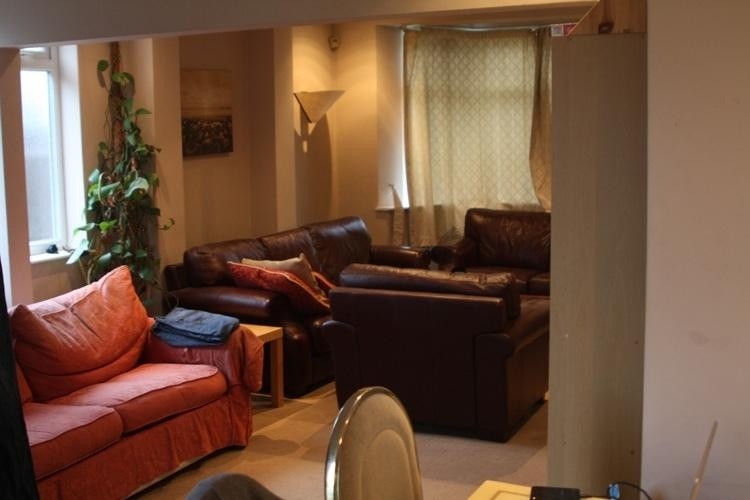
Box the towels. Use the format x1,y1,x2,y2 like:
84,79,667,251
151,307,239,349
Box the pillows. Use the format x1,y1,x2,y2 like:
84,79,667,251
241,251,328,300
225,260,331,315
312,268,337,290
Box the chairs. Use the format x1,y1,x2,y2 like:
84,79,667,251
323,386,424,500
182,471,279,500
313,262,551,444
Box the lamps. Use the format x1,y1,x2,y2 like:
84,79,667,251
294,87,346,122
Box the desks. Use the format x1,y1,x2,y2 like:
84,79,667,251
467,479,532,500
227,322,284,408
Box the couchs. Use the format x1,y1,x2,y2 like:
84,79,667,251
160,214,432,401
434,203,551,295
3,265,265,500
